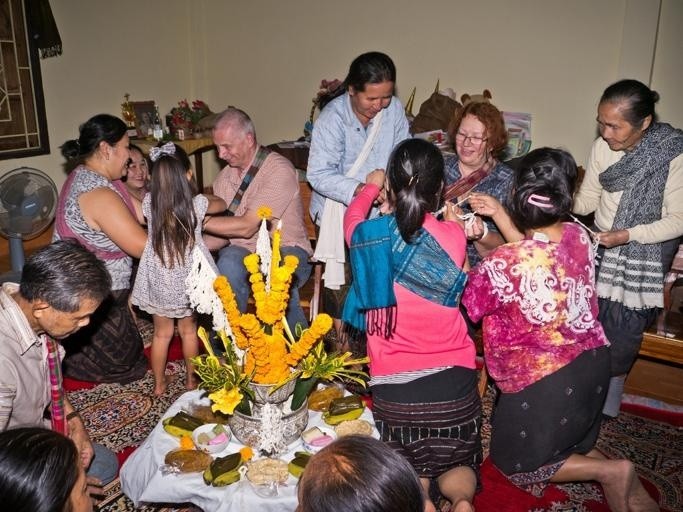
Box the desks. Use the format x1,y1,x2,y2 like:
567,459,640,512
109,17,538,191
130,138,215,194
120,369,382,512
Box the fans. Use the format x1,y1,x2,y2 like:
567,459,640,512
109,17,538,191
0,167,58,286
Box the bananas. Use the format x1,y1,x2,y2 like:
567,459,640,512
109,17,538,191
162,417,208,439
289,451,314,478
203,457,243,486
323,401,366,425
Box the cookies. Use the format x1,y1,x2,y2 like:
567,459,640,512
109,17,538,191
336,420,372,436
245,458,289,485
165,449,214,474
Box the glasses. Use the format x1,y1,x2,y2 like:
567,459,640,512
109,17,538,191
455,132,487,145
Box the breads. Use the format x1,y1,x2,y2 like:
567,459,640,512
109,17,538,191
301,426,323,444
308,386,344,411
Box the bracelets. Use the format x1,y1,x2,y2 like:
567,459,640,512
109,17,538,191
202,214,211,231
66,410,80,421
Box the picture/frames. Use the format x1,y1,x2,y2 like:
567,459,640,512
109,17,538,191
128,100,163,139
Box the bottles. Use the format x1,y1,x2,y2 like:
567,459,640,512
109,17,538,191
151,104,165,142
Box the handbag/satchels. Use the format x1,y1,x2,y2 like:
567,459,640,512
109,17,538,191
410,92,462,136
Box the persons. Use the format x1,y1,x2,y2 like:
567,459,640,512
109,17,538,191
460,148,658,511
200,106,314,369
0,427,93,512
296,434,428,510
344,138,484,511
121,144,179,328
0,237,119,505
52,113,148,385
571,80,682,417
130,141,226,397
306,52,526,393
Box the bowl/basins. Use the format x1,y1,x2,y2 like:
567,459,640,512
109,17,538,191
300,428,338,455
191,422,232,455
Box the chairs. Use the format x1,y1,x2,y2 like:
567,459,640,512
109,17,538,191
468,330,488,398
249,180,323,324
